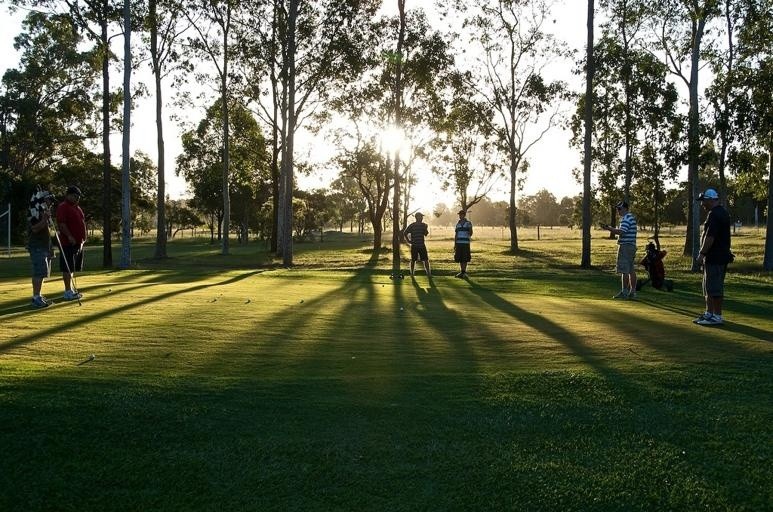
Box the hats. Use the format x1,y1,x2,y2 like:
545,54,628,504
613,201,629,209
415,212,425,217
66,185,85,197
457,210,466,214
695,188,719,201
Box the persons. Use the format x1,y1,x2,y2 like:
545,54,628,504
597,200,640,300
452,210,472,280
21,181,56,308
689,188,737,326
55,186,89,301
403,211,436,277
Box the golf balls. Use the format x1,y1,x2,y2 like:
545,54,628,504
214,298,217,300
109,289,111,291
89,354,94,359
400,307,403,311
301,299,304,302
247,298,251,302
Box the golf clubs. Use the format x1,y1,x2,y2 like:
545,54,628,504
37,183,82,306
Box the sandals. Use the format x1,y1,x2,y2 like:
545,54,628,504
390,273,404,280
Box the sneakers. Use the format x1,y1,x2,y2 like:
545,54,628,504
613,290,635,299
456,272,467,278
692,312,722,326
32,296,49,307
64,290,82,301
